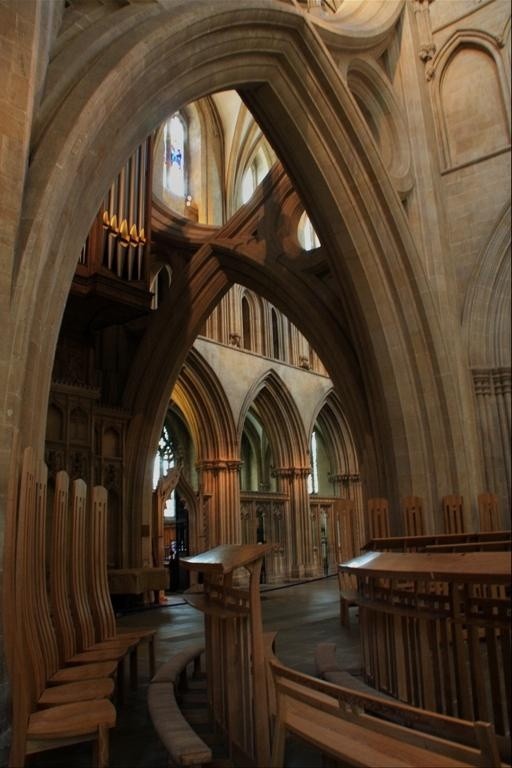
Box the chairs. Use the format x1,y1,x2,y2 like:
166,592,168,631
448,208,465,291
329,500,363,629
15,447,115,700
90,485,157,685
478,493,501,532
55,471,130,694
400,496,426,552
71,478,140,688
35,458,118,686
249,631,501,768
367,497,392,587
443,495,469,534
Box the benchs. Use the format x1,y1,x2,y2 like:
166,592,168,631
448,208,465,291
315,642,339,672
142,644,208,681
147,683,213,767
321,671,403,706
1,441,116,768
